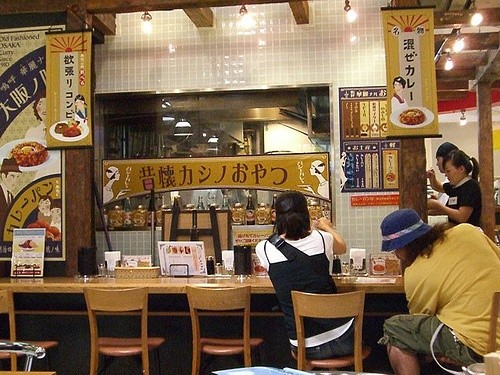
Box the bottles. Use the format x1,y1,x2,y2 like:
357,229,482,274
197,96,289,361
232,203,245,225
342,262,350,275
206,256,214,275
322,204,332,222
255,202,271,225
133,205,146,226
308,202,322,220
196,196,205,210
147,199,157,226
245,194,256,225
220,194,228,210
111,205,124,227
214,263,221,275
332,254,341,274
269,193,277,224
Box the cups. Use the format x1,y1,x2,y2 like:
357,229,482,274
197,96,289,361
78,247,97,277
233,243,252,276
105,251,121,271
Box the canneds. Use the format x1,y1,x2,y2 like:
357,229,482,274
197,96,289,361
307,201,322,227
106,202,274,227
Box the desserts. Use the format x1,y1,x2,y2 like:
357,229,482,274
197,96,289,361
9,141,48,167
399,108,424,125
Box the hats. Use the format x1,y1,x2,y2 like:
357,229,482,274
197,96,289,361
380,208,431,252
436,142,458,157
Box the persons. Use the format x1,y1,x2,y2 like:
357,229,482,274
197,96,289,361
377,208,500,375
255,190,366,361
426,142,483,228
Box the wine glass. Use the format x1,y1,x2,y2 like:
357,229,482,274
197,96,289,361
98,262,106,277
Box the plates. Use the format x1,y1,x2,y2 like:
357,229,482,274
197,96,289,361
390,107,435,128
0,138,59,171
49,119,89,142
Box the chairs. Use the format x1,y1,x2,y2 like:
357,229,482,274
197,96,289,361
427,292,500,375
83,286,166,375
290,290,371,374
0,290,60,372
184,285,264,375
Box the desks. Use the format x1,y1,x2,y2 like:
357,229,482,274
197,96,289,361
0,275,407,317
